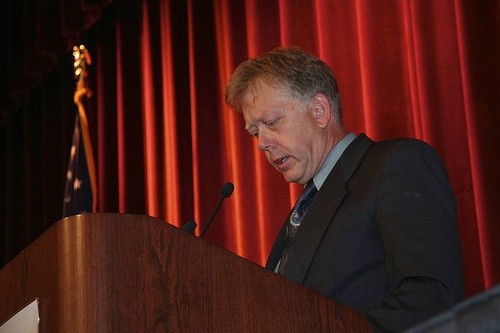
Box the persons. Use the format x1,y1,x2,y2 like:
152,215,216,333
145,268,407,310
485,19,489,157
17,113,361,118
225,46,467,331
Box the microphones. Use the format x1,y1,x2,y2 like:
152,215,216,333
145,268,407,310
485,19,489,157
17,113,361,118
200,181,234,238
180,221,196,233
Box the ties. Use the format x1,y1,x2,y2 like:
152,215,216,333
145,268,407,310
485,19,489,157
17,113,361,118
277,180,318,278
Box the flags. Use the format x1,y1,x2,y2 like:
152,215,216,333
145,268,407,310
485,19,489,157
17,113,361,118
59,109,97,218
